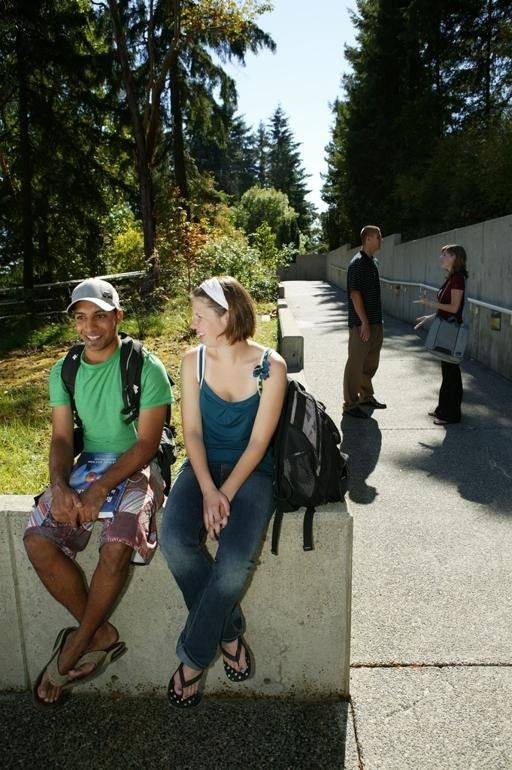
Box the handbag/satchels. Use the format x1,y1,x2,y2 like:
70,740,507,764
425,316,469,368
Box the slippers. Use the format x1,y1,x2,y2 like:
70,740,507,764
64,639,126,692
34,626,80,707
428,410,455,426
166,661,203,709
218,638,253,683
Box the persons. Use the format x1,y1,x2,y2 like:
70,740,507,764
412,245,469,425
23,277,175,708
342,225,386,418
157,274,288,709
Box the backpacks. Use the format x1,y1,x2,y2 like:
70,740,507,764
61,332,179,498
266,376,350,556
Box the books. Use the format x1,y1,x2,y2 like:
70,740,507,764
67,452,130,518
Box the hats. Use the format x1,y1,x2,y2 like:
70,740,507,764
66,278,123,315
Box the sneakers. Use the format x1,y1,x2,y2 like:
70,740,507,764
360,397,387,409
343,401,370,419
